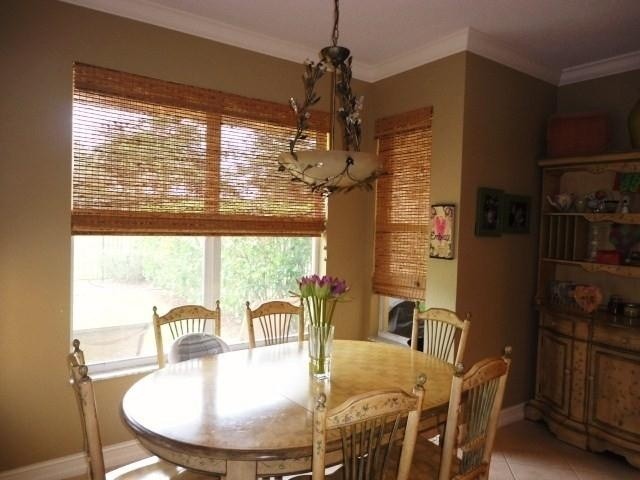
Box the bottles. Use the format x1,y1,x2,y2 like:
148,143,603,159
608,295,639,317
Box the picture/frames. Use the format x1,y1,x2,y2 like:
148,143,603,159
475,187,533,238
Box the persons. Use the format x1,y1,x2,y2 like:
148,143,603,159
511,204,524,232
482,199,497,229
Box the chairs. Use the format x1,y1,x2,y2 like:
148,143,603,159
312,373,427,480
69,338,218,480
410,302,472,368
246,298,304,347
368,345,512,480
151,300,221,369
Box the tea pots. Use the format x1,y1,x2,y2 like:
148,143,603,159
603,199,620,213
546,191,579,213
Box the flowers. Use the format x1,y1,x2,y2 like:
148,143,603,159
290,274,353,375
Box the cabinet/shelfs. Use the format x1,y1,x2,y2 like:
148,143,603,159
524,150,639,469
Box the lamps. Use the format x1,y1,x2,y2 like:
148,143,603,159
273,2,386,199
